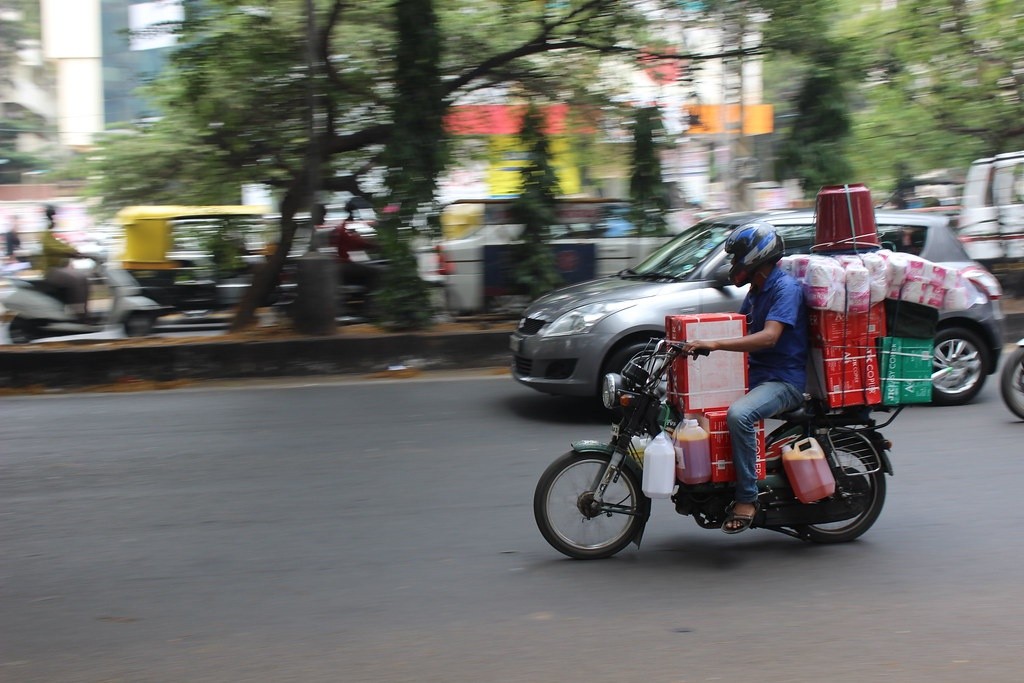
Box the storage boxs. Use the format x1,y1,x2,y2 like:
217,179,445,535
809,300,939,408
666,312,766,482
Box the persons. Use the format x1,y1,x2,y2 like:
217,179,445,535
5,214,21,255
41,204,89,320
302,202,378,264
681,221,810,534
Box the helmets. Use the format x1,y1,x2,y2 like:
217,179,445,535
725,221,785,288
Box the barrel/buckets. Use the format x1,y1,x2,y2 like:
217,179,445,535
672,418,713,481
781,437,835,503
812,182,880,253
641,431,676,499
627,436,652,469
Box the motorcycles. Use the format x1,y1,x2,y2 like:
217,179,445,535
533,335,910,561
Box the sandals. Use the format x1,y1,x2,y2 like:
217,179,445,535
721,498,762,533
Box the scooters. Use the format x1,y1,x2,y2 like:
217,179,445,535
0,238,178,344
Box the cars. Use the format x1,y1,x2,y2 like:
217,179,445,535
508,203,1010,425
